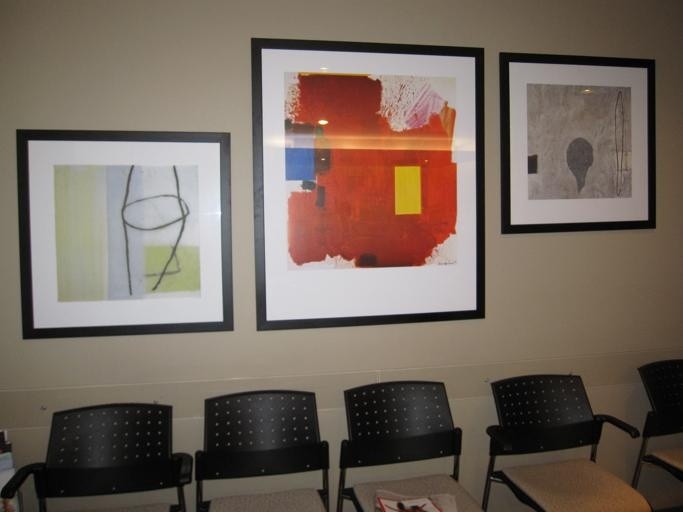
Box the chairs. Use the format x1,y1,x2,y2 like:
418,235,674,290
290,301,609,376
633,359,682,494
2,401,192,512
479,373,655,510
336,380,488,512
194,390,333,510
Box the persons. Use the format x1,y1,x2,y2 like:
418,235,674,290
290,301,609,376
410,503,428,512
385,501,428,512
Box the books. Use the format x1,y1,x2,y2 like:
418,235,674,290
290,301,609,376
375,495,444,512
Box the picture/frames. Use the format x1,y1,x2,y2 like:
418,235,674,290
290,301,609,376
496,48,659,237
13,128,235,339
249,36,488,333
